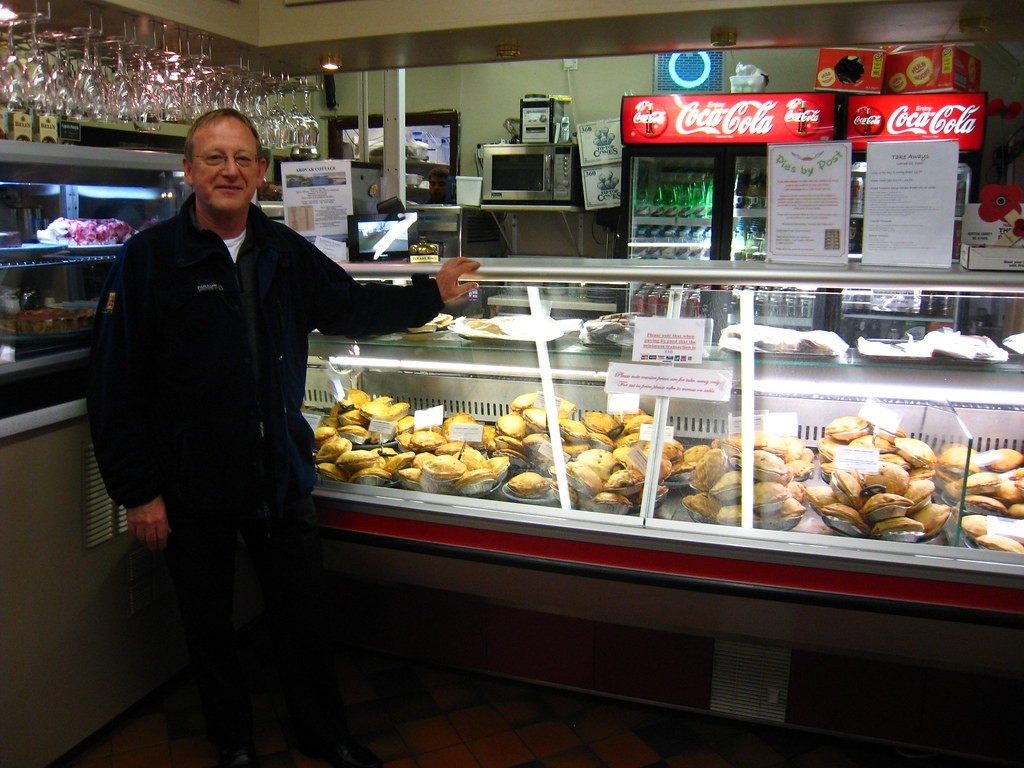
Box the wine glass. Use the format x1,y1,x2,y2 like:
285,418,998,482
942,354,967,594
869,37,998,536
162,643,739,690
0,13,321,150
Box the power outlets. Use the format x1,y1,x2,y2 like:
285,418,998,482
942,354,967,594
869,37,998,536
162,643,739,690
561,58,579,71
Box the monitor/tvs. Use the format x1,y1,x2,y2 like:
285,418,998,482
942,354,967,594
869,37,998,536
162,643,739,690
347,211,418,262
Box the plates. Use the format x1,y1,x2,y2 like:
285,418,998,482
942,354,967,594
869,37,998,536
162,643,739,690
39,240,124,253
0,243,66,261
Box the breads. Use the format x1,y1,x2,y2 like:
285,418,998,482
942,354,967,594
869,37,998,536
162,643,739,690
313,388,1024,554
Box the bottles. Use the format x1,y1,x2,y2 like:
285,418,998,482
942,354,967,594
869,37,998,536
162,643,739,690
634,172,713,218
634,224,712,259
852,294,899,349
732,217,761,261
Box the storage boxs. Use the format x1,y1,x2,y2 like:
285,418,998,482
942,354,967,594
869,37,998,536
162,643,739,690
814,47,888,94
729,75,766,94
887,46,982,96
960,243,1024,271
455,175,483,207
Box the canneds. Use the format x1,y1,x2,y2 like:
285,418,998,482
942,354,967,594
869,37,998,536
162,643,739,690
955,181,966,217
731,286,811,319
851,177,864,214
633,286,699,318
849,220,858,253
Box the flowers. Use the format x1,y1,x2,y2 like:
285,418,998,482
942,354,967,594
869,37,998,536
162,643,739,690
986,99,1022,183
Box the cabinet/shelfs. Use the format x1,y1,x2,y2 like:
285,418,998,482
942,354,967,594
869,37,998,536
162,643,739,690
303,261,1024,768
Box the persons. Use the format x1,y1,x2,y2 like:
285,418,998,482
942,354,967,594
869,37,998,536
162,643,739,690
421,167,447,205
84,109,478,768
899,301,953,341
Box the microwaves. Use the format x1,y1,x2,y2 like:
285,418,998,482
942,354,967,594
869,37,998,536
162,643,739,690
481,140,575,202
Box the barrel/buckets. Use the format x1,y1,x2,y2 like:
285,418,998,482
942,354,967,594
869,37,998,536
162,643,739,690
455,175,483,207
